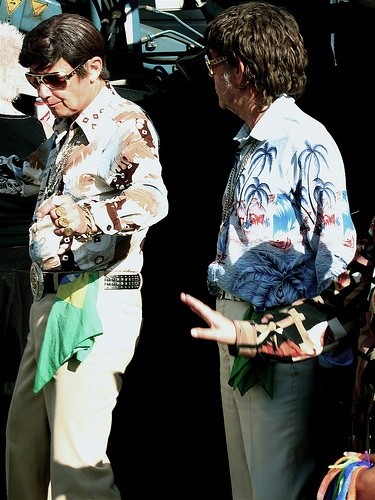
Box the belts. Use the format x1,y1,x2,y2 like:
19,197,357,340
30,262,140,301
218,290,243,301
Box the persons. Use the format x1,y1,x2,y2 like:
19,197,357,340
180,217,375,460
356,454,375,500
204,1,357,500
5,13,170,500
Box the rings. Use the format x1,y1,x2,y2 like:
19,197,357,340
51,195,74,243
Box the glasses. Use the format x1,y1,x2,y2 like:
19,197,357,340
205,54,229,75
25,56,92,91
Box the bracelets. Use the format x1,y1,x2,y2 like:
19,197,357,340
74,204,93,243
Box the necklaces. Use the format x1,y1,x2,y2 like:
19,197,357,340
222,139,260,227
45,126,85,199
